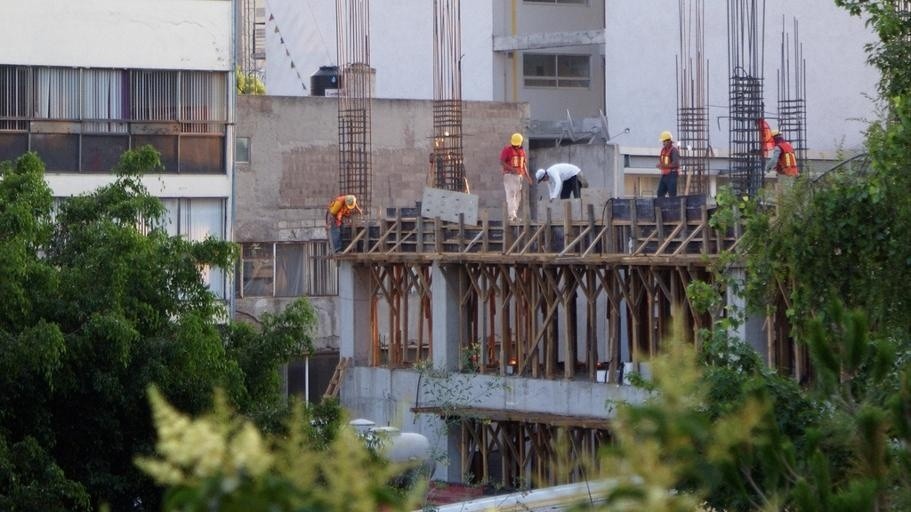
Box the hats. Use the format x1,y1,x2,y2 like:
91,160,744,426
345,193,357,211
771,129,782,136
536,168,546,184
511,133,525,148
659,131,672,141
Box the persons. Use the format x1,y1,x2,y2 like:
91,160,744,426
765,130,797,192
535,163,589,203
501,133,533,224
757,118,775,157
656,132,680,198
325,194,362,255
